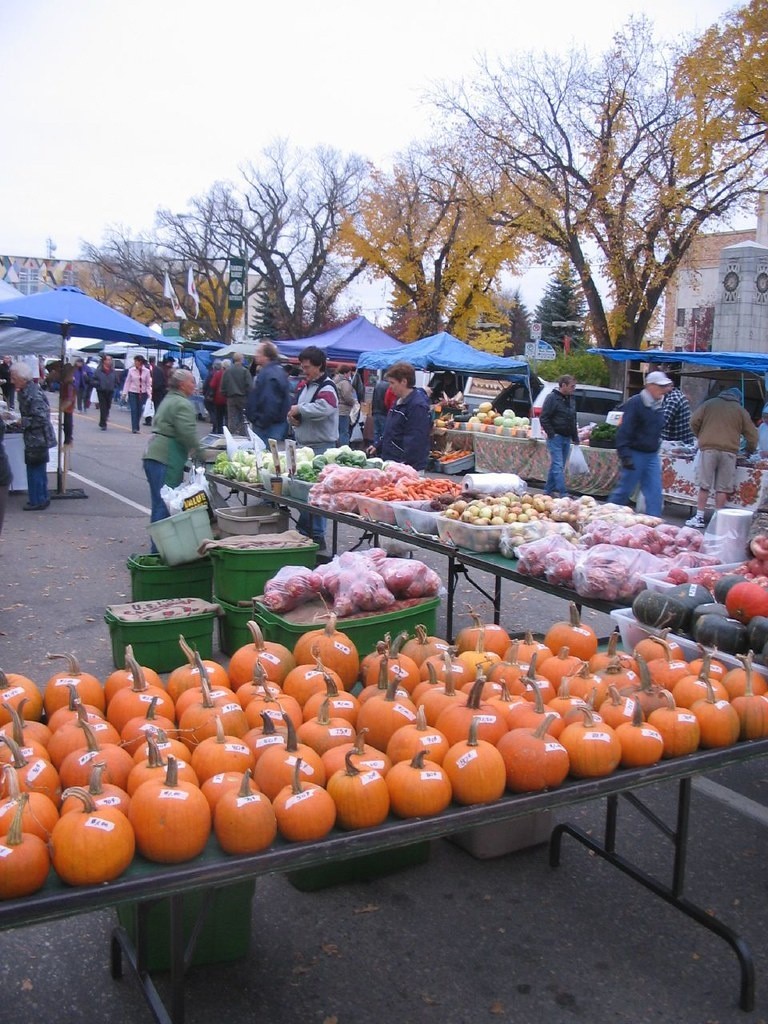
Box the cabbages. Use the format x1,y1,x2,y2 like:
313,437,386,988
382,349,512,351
493,409,529,428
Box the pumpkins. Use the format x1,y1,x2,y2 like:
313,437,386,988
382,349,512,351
0,600,768,901
632,574,768,668
440,450,470,464
454,401,495,425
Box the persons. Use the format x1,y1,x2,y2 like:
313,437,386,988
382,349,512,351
287,346,339,550
756,403,768,458
72,358,91,413
685,388,760,527
6,362,56,510
541,376,579,498
143,357,175,426
203,352,253,434
662,383,694,447
122,355,153,434
60,363,75,450
247,342,291,510
0,356,14,410
333,365,363,450
606,371,672,519
90,355,120,430
365,362,462,474
0,419,13,533
143,369,205,554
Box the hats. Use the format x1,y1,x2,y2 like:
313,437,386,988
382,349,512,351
646,371,672,386
763,406,768,414
730,387,742,401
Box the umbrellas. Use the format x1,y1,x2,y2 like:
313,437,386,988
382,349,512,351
77,323,256,365
588,349,768,397
0,285,182,501
349,332,534,416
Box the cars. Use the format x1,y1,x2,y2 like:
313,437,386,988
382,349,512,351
111,357,125,371
41,357,61,373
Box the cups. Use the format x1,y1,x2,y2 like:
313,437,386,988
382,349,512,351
270,477,283,496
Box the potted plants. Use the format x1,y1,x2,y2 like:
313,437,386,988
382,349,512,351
588,422,619,449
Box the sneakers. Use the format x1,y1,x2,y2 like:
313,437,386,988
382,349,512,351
685,516,706,527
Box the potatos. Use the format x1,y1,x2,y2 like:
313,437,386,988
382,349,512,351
516,520,721,601
663,535,768,595
261,548,442,616
419,491,491,512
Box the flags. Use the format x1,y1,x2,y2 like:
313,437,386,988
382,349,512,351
164,275,188,320
188,269,200,318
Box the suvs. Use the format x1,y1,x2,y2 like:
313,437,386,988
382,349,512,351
489,355,625,431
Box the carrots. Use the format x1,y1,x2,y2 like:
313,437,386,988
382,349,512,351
309,464,463,523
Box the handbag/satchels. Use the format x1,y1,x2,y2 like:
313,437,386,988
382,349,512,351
223,426,266,462
25,415,50,465
370,533,419,553
143,398,155,418
350,422,364,442
636,489,665,514
89,387,99,403
161,466,219,526
569,444,588,476
349,402,360,425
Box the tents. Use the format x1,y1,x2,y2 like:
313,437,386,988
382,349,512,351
276,318,403,362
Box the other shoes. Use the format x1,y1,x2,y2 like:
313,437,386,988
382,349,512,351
558,494,577,500
543,492,559,498
23,500,50,510
388,551,411,559
102,426,106,431
311,536,327,551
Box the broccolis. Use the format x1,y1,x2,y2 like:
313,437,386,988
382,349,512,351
367,457,383,463
222,444,366,482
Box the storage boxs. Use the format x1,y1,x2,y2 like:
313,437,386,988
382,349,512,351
260,469,289,495
434,459,443,472
287,478,317,502
389,503,439,535
351,492,395,525
440,452,475,474
445,811,552,860
103,507,440,663
452,421,525,436
609,608,768,679
640,562,742,594
432,512,502,554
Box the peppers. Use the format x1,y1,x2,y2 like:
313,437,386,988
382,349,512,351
296,452,383,482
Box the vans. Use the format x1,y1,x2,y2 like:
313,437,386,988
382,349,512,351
678,366,768,429
286,355,512,415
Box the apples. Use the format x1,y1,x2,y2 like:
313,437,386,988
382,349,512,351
445,491,663,559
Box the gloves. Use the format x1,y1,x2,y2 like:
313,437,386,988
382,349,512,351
622,457,636,470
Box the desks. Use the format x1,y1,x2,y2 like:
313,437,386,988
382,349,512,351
431,428,768,520
1,412,29,492
45,412,65,473
184,459,640,643
0,736,768,1024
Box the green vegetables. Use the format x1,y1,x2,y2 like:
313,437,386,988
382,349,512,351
590,421,619,440
214,450,228,475
